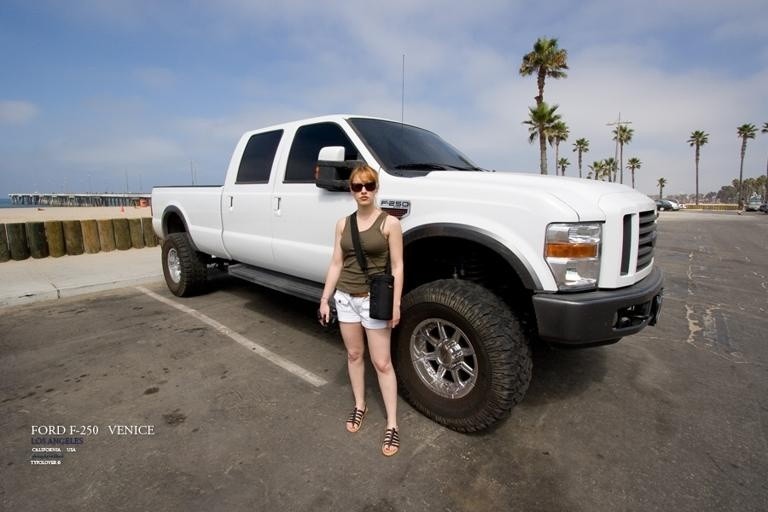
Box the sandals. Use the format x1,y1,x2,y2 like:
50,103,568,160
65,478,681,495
346,402,368,433
381,424,399,457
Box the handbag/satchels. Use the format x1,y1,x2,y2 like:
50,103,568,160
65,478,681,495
370,274,394,320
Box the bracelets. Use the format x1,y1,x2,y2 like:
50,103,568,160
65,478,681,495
319,296,328,305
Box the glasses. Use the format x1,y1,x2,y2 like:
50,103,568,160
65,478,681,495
350,180,377,192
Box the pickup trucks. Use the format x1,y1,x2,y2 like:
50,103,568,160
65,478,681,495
148,113,663,436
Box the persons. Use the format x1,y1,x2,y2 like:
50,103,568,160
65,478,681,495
736,197,744,216
315,163,404,457
